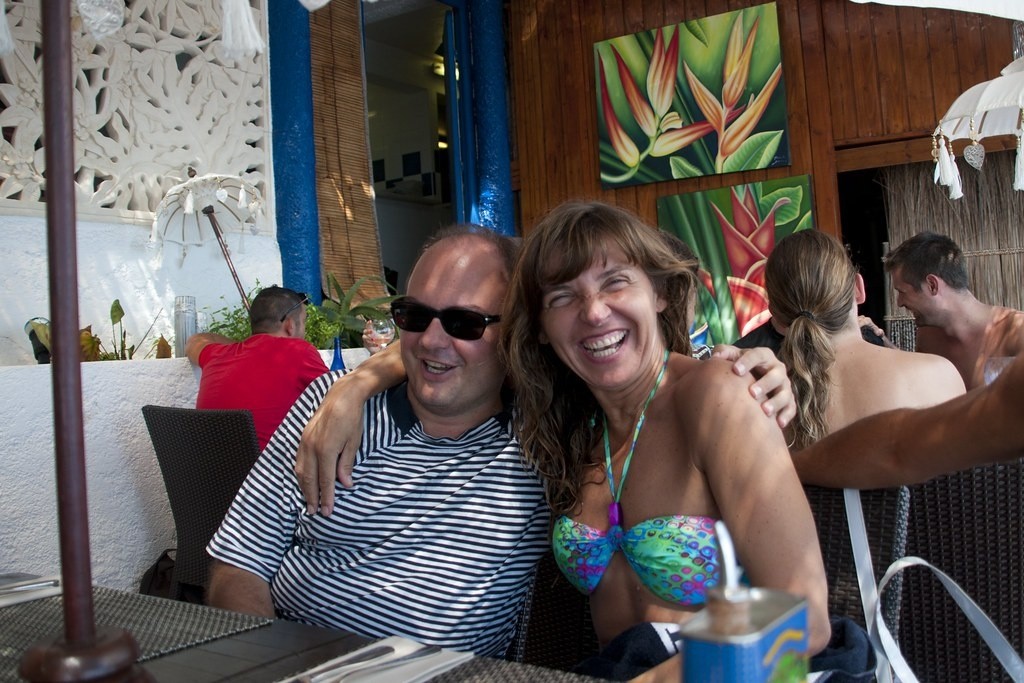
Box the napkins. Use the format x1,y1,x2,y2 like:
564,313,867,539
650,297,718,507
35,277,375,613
278,635,475,683
0,574,62,607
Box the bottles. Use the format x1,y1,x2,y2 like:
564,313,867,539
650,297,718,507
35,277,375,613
329,336,346,371
174,297,198,359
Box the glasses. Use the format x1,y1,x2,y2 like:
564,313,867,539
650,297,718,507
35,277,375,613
281,292,308,322
390,298,503,341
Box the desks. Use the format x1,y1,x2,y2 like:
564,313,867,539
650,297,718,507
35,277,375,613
0,582,357,683
218,632,610,683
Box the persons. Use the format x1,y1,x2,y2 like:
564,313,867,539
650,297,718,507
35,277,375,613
764,228,967,444
185,286,333,455
294,200,831,683
855,233,1024,393
206,221,798,661
788,346,1024,490
729,318,884,356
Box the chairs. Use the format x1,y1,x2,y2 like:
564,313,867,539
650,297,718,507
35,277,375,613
900,460,1024,683
141,404,261,601
525,483,911,671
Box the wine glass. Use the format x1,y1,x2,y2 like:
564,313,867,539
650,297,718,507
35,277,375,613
369,320,396,355
